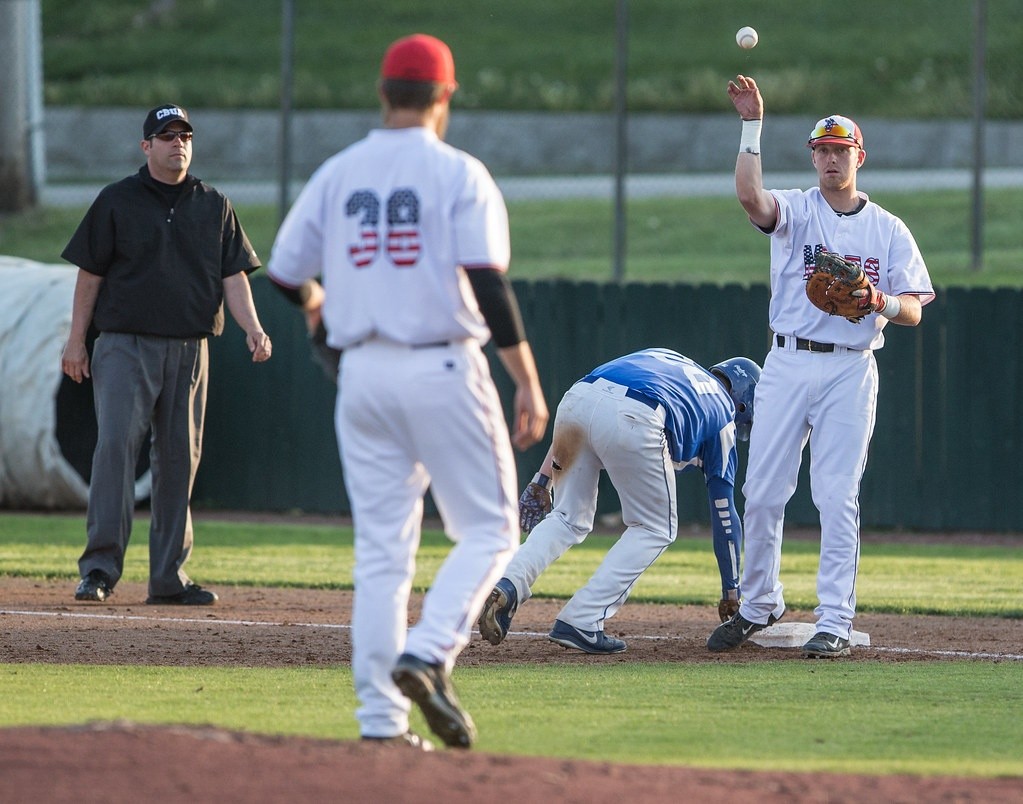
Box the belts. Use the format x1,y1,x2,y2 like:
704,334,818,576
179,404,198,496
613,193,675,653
777,335,855,352
580,374,660,411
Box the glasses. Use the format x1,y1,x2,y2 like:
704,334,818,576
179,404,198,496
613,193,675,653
146,130,194,140
807,125,861,148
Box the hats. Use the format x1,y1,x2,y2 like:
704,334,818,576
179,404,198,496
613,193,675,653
144,105,193,141
806,115,863,149
383,33,458,85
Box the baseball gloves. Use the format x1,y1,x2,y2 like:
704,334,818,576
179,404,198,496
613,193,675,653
805,250,877,325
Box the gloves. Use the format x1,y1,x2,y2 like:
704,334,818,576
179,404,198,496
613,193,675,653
718,599,740,622
519,472,552,534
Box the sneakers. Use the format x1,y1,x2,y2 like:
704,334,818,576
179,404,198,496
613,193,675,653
147,584,218,605
548,620,627,654
363,729,436,750
478,579,517,646
802,632,852,659
390,653,477,750
75,570,111,601
708,611,783,652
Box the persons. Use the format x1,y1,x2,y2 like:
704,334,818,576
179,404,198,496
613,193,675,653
266,33,550,753
60,104,272,605
706,74,935,659
477,348,763,652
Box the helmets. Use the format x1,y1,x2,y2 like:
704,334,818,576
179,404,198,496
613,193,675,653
708,357,762,441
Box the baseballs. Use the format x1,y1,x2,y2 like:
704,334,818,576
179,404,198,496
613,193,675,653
735,25,759,49
